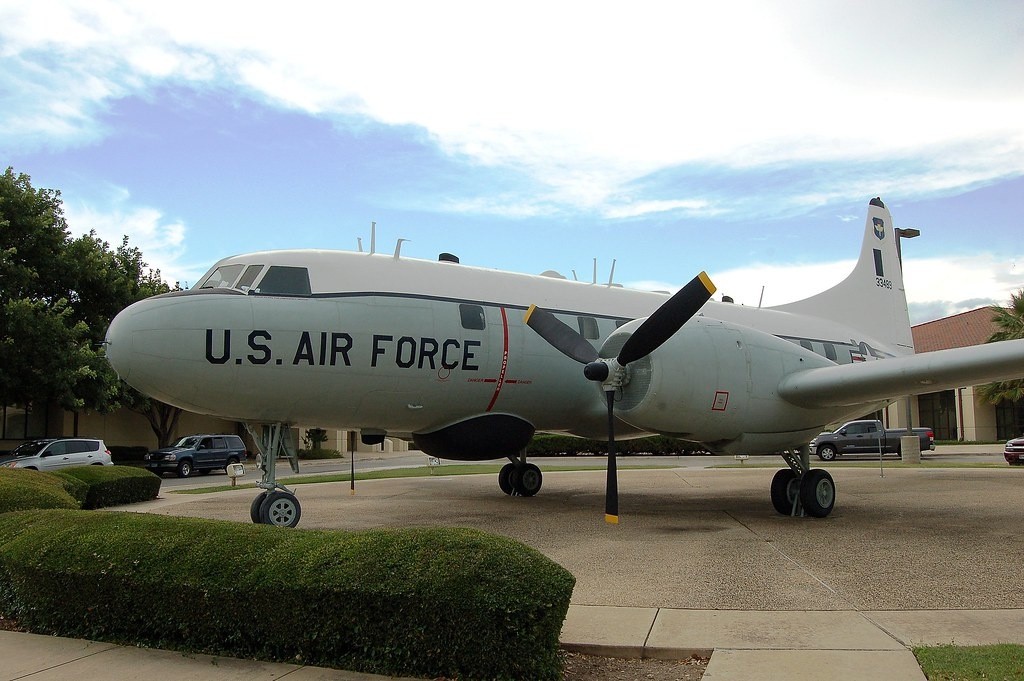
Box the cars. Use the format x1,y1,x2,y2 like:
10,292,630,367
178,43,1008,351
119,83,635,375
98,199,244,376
1004,436,1024,466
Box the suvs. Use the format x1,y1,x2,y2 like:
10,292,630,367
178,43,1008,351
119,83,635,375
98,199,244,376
143,434,248,478
0,437,115,473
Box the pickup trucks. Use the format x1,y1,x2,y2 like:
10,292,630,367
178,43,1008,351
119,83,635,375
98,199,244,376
808,420,935,462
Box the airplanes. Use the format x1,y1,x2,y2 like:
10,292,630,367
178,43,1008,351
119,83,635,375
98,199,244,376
101,192,1024,528
